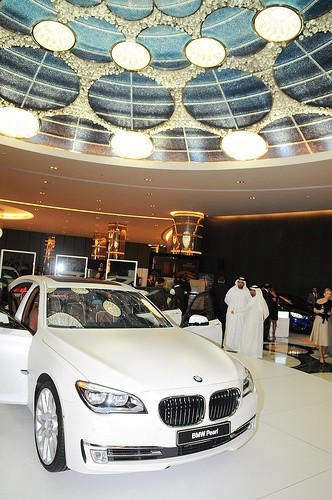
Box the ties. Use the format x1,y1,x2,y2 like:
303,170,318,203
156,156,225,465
315,293,317,298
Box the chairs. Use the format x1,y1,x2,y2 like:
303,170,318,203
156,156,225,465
13,293,131,328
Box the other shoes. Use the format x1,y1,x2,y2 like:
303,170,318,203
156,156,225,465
264,339,272,342
319,356,325,366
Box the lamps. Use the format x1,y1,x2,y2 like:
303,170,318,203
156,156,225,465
90,234,107,260
106,222,127,259
171,210,204,254
44,237,56,258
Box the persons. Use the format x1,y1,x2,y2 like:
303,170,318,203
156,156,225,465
267,288,279,340
97,263,106,277
307,287,320,308
137,277,142,286
95,272,100,279
224,277,250,352
167,278,187,311
231,285,269,358
262,284,271,342
158,278,165,286
154,280,159,286
182,276,191,314
309,288,332,363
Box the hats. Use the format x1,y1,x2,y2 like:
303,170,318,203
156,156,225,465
235,277,246,290
250,285,262,297
